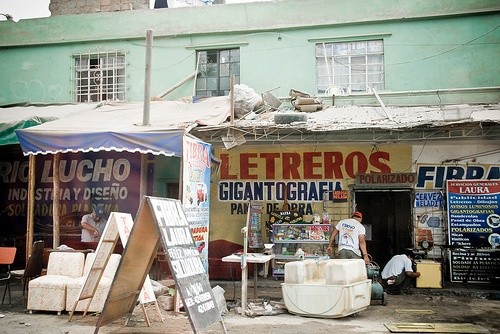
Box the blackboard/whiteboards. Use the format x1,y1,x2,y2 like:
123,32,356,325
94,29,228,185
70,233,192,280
148,198,221,330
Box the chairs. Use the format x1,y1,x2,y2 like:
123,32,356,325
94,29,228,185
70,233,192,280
0,247,17,308
28,252,121,315
1,240,44,308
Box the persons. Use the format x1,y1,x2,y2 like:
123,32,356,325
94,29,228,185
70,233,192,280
80,206,107,252
326,211,370,265
380,250,420,295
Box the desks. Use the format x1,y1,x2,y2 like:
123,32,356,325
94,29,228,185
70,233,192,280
222,251,275,299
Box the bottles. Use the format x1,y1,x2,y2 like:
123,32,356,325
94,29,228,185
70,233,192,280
313,210,320,224
322,211,329,224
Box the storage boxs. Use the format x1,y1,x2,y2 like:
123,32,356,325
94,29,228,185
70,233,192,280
414,259,443,289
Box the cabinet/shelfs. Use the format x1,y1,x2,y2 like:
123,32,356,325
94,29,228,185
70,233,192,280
269,211,333,281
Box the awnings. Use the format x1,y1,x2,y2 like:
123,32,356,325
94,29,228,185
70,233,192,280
0,87,500,157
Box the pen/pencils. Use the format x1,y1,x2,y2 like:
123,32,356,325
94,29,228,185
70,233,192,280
252,254,255,257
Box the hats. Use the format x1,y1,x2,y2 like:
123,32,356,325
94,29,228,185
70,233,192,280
351,211,363,218
94,206,106,219
